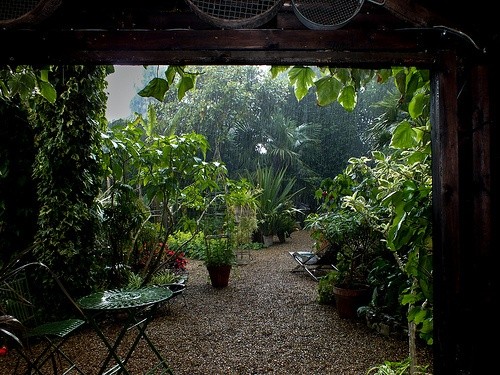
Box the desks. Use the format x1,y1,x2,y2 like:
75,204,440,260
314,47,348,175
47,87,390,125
78,286,173,375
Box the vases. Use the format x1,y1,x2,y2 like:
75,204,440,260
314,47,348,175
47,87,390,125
332,275,364,320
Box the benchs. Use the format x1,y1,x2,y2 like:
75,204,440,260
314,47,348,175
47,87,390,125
287,240,342,283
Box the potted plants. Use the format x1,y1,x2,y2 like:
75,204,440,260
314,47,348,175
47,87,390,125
207,244,233,288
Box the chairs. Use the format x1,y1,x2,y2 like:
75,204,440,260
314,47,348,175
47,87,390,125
0,272,85,375
